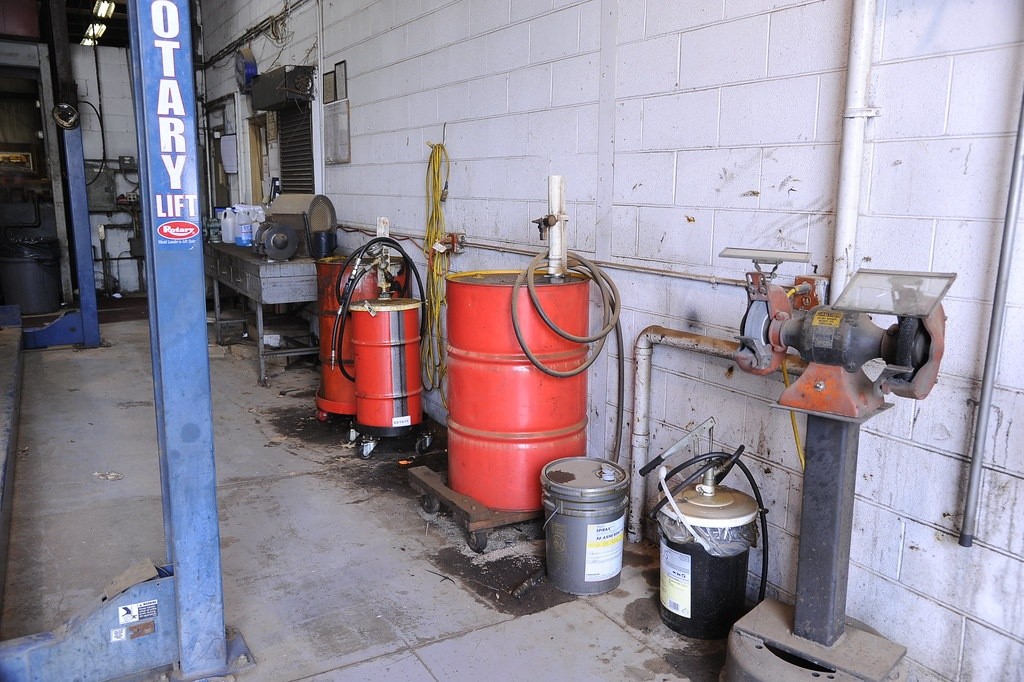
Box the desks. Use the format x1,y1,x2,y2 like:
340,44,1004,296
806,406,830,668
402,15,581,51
203,242,320,384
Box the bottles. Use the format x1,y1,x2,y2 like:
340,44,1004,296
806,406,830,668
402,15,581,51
220,205,266,247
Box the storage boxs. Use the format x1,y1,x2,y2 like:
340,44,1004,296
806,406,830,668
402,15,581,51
247,318,310,346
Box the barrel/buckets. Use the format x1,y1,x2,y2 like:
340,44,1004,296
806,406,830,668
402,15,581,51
541,456,629,595
445,270,589,513
347,297,422,438
659,484,759,638
315,256,412,415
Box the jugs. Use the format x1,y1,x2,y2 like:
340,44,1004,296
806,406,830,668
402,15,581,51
302,212,337,260
